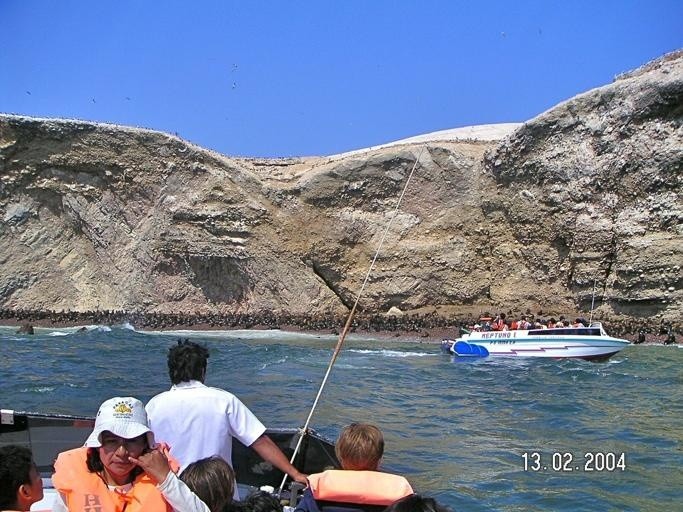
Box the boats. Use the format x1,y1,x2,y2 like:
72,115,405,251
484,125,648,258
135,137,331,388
441,320,631,361
0,409,343,500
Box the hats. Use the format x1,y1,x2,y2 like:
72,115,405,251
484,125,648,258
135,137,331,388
82,396,160,450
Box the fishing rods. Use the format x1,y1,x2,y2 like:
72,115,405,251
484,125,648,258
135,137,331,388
277,141,426,496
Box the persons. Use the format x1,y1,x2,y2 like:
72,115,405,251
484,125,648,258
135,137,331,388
475,313,586,331
